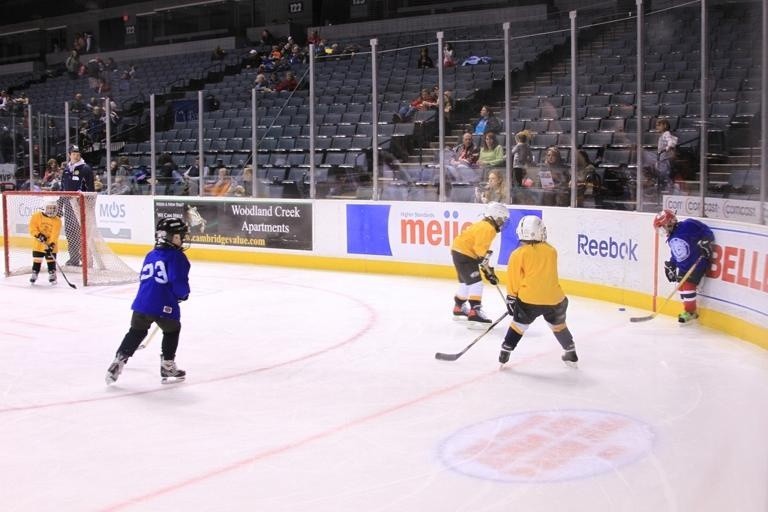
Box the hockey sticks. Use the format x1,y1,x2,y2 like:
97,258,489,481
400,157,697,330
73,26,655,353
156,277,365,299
435,311,509,360
137,325,160,350
630,255,703,322
36,227,77,288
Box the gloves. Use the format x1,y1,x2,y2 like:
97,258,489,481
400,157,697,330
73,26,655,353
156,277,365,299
697,239,714,261
664,257,678,284
484,266,500,285
505,295,518,316
47,242,56,252
38,232,47,243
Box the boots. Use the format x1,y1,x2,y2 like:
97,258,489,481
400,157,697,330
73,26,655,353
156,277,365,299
466,299,492,324
561,350,578,362
454,296,470,317
160,354,187,378
498,341,513,364
678,310,700,323
48,269,57,282
107,352,128,381
29,270,38,282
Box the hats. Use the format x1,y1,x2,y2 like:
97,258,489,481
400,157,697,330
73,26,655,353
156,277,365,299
68,144,82,154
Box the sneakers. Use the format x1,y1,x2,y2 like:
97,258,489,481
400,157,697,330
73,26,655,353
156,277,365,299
66,258,80,265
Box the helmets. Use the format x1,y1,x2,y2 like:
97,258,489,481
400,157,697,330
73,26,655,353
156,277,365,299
482,200,511,230
654,209,678,234
42,198,58,214
155,217,188,251
516,215,548,243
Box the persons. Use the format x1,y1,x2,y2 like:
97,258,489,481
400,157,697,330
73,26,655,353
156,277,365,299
450,203,510,330
444,42,456,68
390,84,455,135
449,132,602,210
417,47,434,68
105,218,187,386
468,104,500,134
642,118,678,195
497,216,578,368
652,208,715,329
27,200,62,287
60,144,96,270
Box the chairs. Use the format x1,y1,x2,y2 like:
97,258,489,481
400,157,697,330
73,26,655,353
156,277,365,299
0,0,767,210
